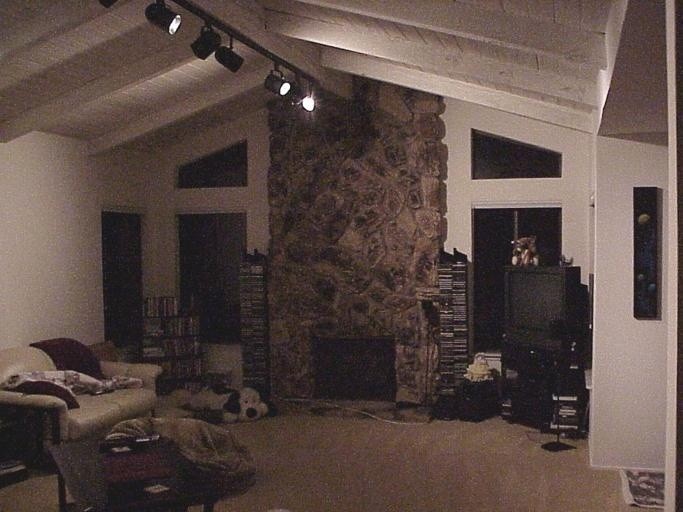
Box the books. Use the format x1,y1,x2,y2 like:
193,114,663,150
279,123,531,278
435,261,468,394
548,387,579,430
141,261,270,388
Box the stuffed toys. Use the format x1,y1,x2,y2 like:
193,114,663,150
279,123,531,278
178,378,278,427
510,235,540,267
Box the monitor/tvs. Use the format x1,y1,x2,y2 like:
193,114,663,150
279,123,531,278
504,265,581,332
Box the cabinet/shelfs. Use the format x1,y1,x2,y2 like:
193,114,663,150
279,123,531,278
140,296,206,395
498,336,590,440
438,247,469,392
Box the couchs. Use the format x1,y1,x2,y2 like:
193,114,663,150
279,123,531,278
1,336,164,472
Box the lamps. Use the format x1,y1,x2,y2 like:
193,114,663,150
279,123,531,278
145,0,321,113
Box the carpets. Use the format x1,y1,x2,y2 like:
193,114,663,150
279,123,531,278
621,469,666,511
